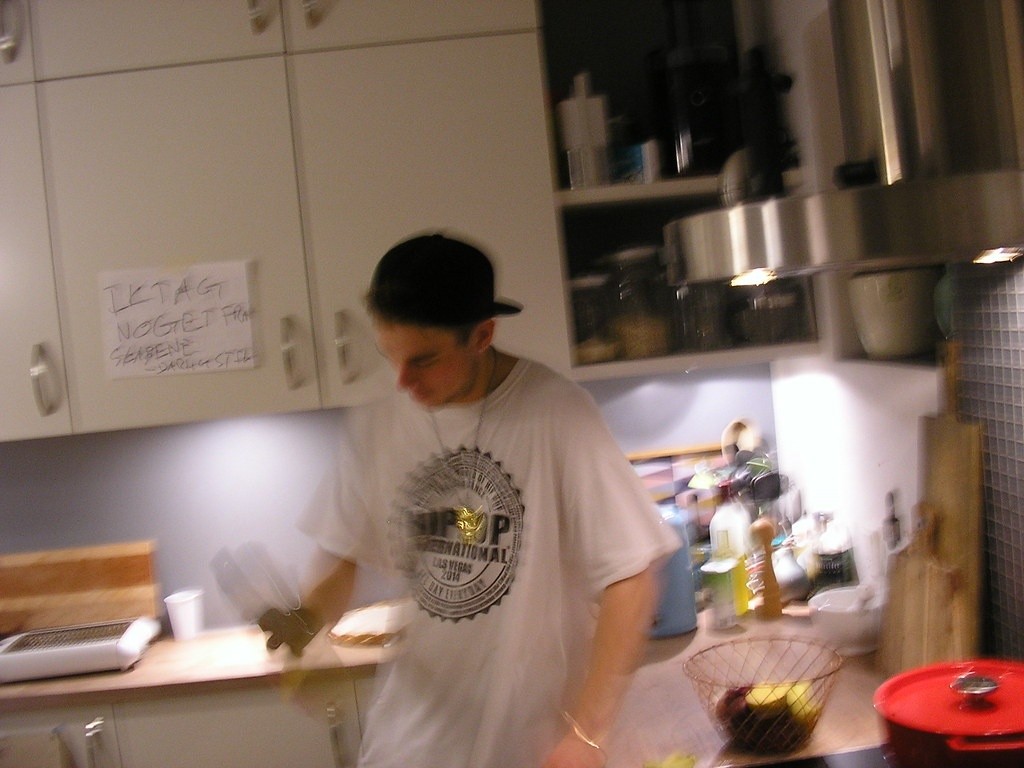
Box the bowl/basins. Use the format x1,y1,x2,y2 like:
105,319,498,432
844,254,948,357
808,587,884,657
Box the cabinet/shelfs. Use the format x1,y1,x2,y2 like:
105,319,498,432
537,0,829,382
112,675,373,768
1,0,73,441
28,0,574,434
0,703,122,768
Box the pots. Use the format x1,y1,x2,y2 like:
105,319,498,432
873,657,1024,768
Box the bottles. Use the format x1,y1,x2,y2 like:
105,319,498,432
569,246,802,364
773,549,809,604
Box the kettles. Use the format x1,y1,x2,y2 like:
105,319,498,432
648,512,699,636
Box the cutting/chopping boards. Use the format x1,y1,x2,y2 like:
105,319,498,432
880,414,981,677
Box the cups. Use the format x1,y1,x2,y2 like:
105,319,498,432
161,590,202,640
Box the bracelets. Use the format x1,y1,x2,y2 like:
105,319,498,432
287,610,318,637
560,711,604,751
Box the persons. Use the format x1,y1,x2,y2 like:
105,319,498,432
257,232,682,768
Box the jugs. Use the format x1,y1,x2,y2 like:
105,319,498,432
556,67,617,190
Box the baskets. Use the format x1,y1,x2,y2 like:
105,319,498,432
683,634,843,753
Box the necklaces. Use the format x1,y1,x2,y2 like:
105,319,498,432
425,343,498,509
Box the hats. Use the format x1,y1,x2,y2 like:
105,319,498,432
368,234,523,324
